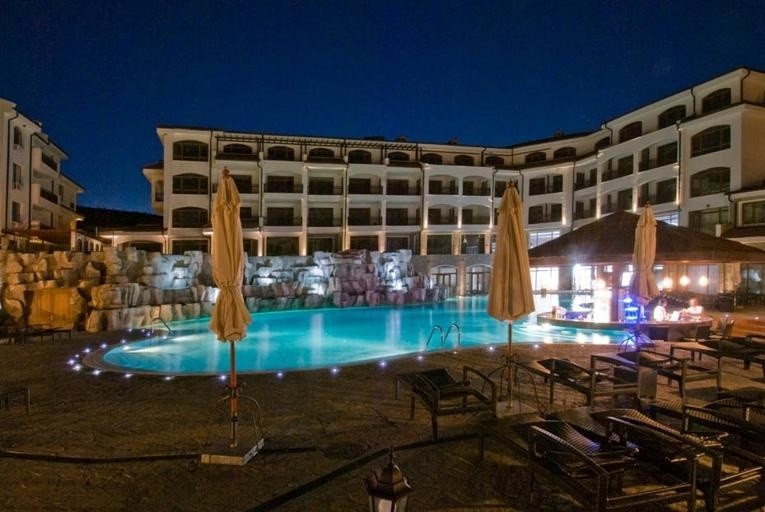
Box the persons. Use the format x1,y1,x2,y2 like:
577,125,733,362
653,297,673,321
682,298,705,320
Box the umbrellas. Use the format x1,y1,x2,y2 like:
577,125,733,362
627,200,661,348
206,164,255,449
487,177,537,411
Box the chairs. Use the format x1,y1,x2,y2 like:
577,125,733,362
0,323,72,344
